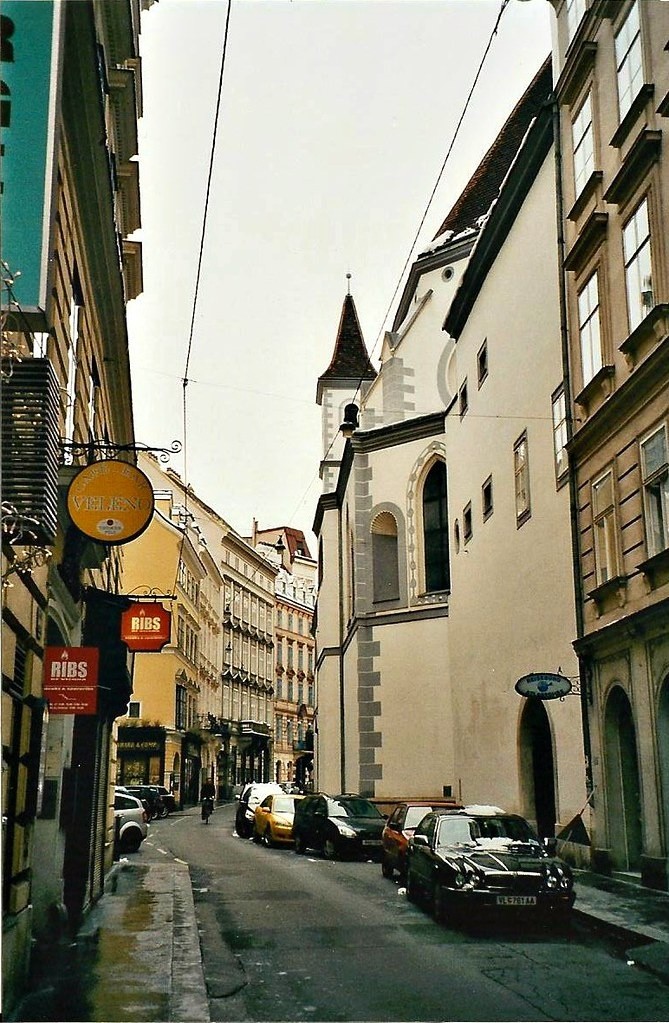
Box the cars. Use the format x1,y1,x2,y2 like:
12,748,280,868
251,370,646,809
381,801,466,885
407,810,576,925
254,795,308,847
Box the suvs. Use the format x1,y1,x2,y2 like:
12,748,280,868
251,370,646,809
235,782,279,800
113,786,148,854
236,787,289,838
126,785,175,820
292,792,389,861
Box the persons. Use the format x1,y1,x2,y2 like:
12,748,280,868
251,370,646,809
200,777,215,820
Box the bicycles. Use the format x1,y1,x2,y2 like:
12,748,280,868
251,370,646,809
202,796,214,825
